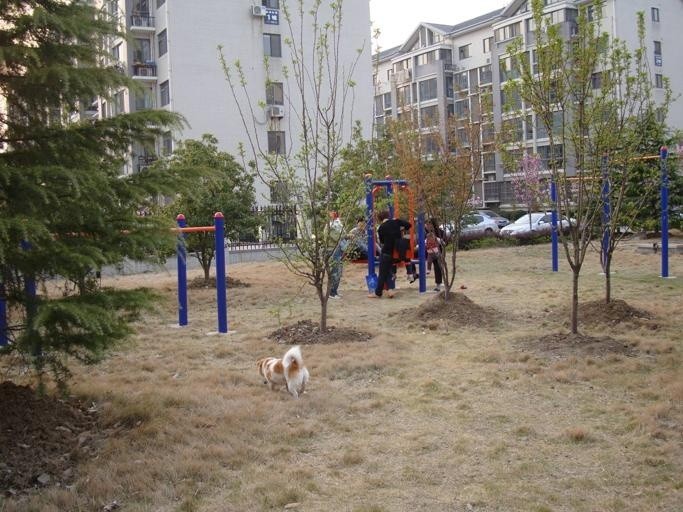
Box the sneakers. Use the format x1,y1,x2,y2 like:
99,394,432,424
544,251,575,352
337,293,343,297
328,294,340,299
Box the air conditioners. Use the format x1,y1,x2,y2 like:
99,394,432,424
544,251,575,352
268,105,284,119
252,4,266,17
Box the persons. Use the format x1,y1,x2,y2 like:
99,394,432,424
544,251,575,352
345,208,449,300
327,210,349,261
322,209,355,298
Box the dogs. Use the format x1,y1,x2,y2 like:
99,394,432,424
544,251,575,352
256,346,309,400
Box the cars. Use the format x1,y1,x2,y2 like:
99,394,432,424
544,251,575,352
469,209,509,227
438,213,499,243
498,212,578,240
